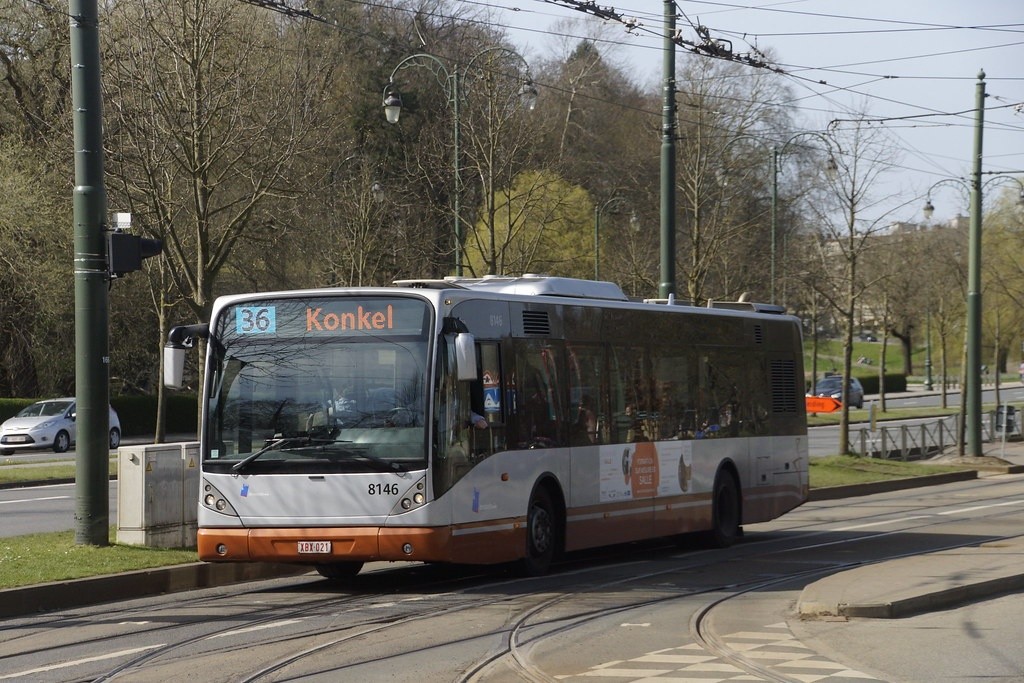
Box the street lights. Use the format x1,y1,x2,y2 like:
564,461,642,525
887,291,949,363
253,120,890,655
918,173,1023,454
592,196,642,281
716,131,840,307
380,42,541,278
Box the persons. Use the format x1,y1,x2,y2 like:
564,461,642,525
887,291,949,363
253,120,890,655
382,386,488,440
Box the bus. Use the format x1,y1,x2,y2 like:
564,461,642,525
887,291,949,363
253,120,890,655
164,272,814,580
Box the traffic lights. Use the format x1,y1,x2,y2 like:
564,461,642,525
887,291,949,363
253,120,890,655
109,233,165,274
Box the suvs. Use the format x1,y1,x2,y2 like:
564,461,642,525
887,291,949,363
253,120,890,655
809,372,864,409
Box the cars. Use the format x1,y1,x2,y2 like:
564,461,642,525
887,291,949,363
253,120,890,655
0,395,124,454
